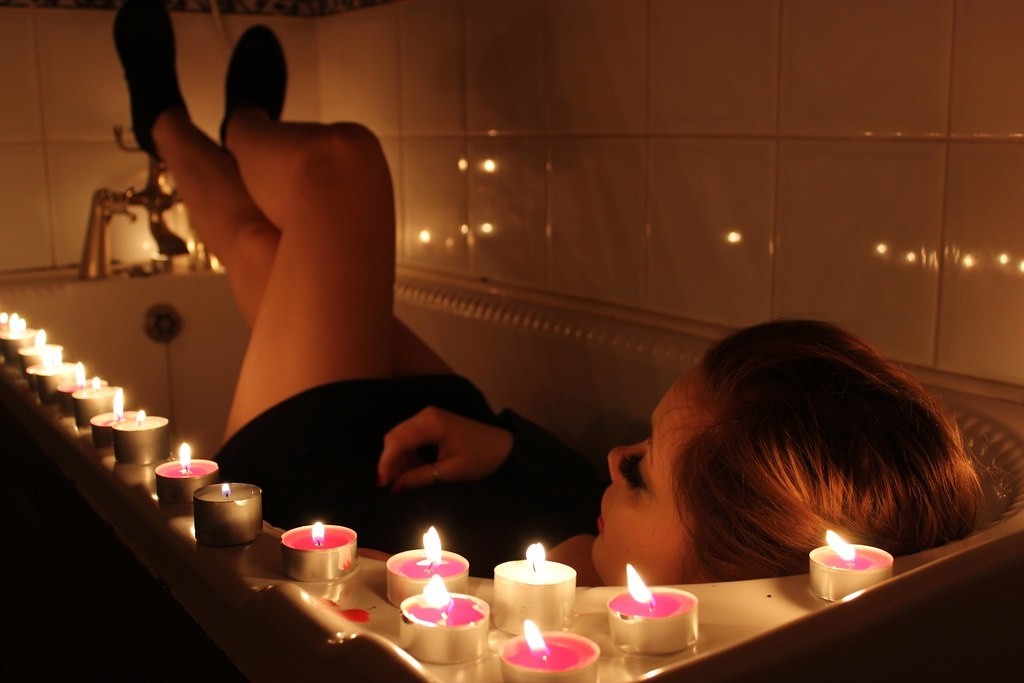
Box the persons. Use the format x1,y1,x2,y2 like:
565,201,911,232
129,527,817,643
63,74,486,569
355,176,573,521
112,0,985,589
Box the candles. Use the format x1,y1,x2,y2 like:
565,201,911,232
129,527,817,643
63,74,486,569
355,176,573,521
608,587,699,652
385,548,469,607
400,592,489,662
499,631,600,683
155,460,220,503
810,545,894,601
494,561,577,636
192,483,262,546
280,524,358,580
0,312,173,466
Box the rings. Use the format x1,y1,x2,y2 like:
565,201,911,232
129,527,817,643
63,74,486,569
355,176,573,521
432,462,440,482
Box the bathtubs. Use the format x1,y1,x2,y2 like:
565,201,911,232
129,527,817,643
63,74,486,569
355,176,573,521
1,253,1024,683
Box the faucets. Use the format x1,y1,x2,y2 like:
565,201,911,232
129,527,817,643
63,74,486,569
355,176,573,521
82,185,146,276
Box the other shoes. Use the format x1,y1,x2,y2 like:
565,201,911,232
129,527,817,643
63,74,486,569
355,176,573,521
220,25,288,141
112,0,189,159
